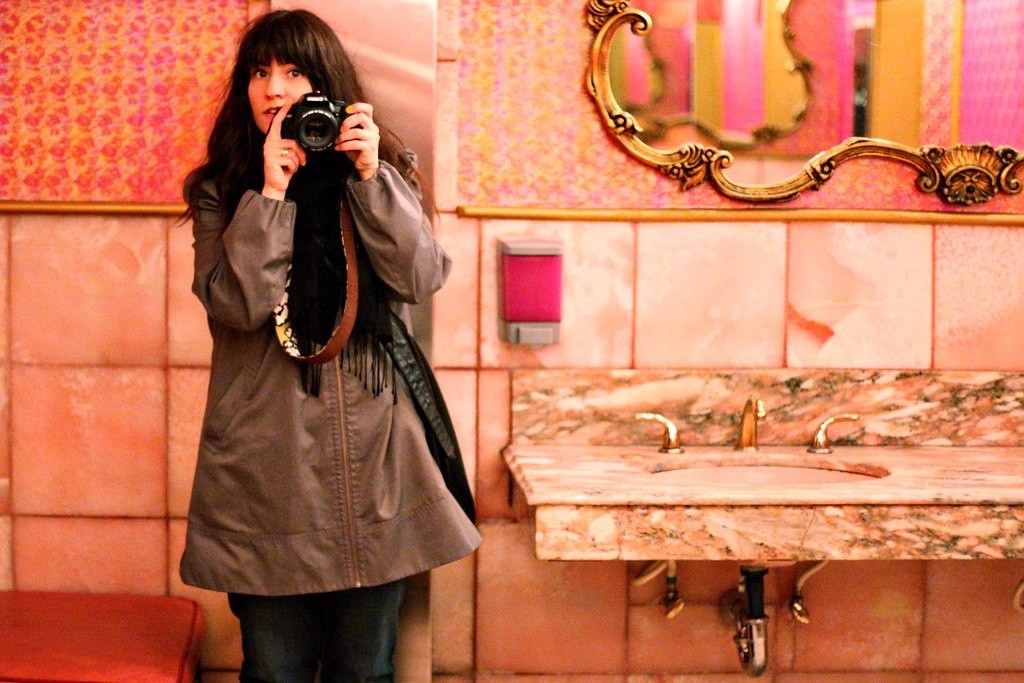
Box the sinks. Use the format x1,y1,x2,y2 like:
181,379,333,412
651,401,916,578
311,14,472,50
641,453,893,485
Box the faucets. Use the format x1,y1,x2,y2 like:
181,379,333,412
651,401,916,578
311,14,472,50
732,393,768,453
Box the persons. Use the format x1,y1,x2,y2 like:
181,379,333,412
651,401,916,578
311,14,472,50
179,8,482,683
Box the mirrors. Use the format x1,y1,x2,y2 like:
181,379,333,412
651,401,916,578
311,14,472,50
577,2,1024,206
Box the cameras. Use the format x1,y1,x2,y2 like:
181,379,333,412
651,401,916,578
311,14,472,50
281,91,362,153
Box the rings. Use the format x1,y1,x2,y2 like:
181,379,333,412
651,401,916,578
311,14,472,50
281,146,289,156
